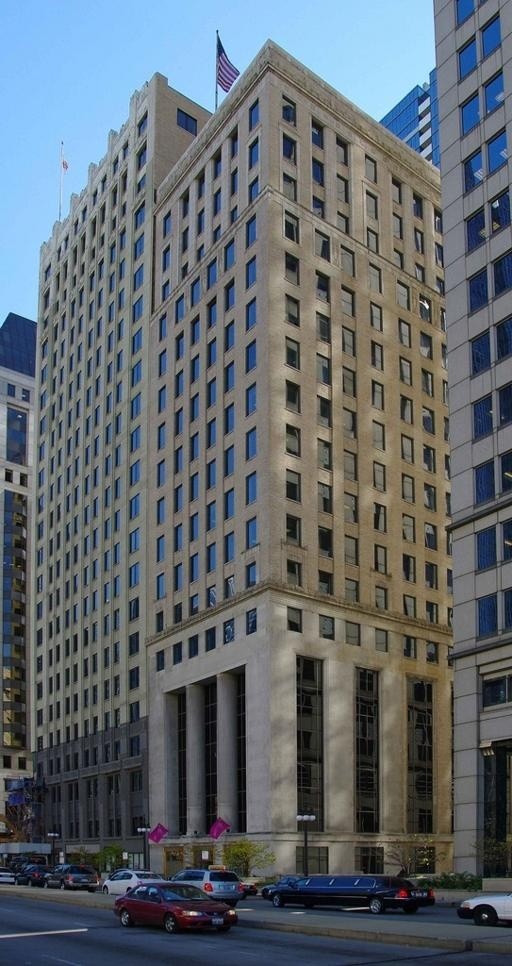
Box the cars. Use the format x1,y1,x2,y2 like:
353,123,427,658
458,891,512,926
0,864,100,893
262,874,434,914
102,867,166,895
241,880,257,900
116,882,238,933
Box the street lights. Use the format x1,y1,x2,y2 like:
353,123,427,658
296,812,316,874
137,822,151,868
48,832,59,864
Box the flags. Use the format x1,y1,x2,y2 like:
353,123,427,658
208,816,233,840
58,144,71,176
215,32,243,95
148,822,170,845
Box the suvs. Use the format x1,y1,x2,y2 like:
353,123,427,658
170,866,244,905
